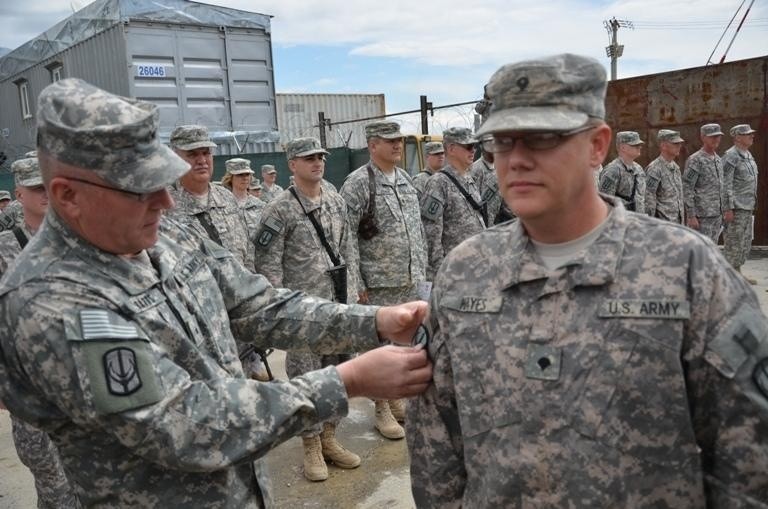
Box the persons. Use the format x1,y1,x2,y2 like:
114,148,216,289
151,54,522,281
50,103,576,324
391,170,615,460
1,147,37,236
2,189,11,213
681,123,725,245
644,128,686,226
720,122,759,287
0,76,433,509
599,129,647,214
161,121,516,484
1,158,80,508
402,51,767,509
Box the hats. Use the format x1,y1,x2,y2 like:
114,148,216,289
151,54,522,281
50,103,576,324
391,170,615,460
10,157,48,189
0,190,12,203
424,141,446,156
24,150,38,158
223,156,255,177
284,136,333,161
244,176,265,191
614,130,646,146
260,164,279,176
729,123,757,138
654,129,686,144
473,52,610,136
698,123,726,138
170,125,218,152
34,75,193,195
438,127,481,146
363,120,411,141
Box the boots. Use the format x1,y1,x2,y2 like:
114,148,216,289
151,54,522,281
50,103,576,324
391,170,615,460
737,267,757,285
372,399,407,440
319,421,361,469
300,435,330,482
387,395,410,422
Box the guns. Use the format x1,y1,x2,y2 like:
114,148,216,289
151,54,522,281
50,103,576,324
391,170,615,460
326,261,349,364
239,344,277,382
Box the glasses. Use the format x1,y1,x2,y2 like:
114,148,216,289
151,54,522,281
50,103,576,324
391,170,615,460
477,125,598,154
137,191,161,205
